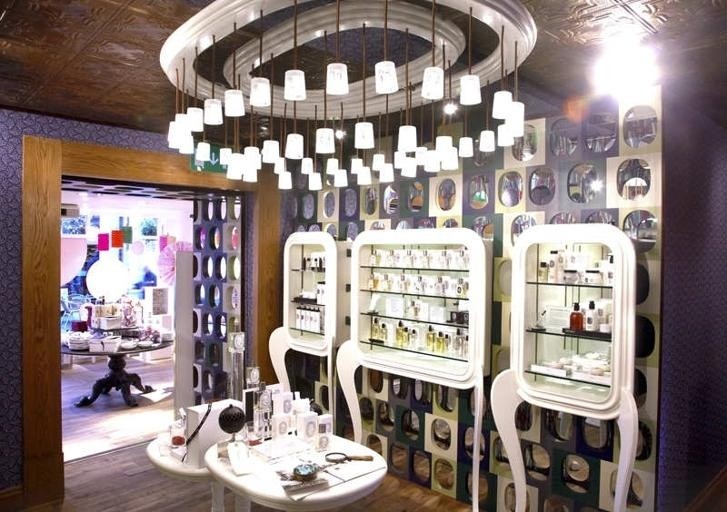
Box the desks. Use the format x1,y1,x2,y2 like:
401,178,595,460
59,328,174,409
204,430,389,511
145,415,263,512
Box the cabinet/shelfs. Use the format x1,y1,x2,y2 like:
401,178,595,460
489,224,644,512
267,228,347,434
334,227,492,512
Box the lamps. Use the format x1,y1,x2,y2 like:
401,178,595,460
489,23,513,117
247,8,274,109
416,102,428,166
457,108,475,158
109,231,126,249
261,53,282,166
457,5,483,107
423,100,443,173
372,112,386,172
277,156,294,191
324,0,352,97
441,116,459,172
121,224,136,244
378,94,395,184
436,103,455,150
219,118,235,168
195,126,213,162
324,154,339,175
373,0,401,96
201,34,225,127
95,232,111,253
284,101,305,161
178,132,193,156
506,40,527,139
241,64,262,170
400,83,420,179
479,80,496,153
331,101,345,189
396,28,418,153
313,69,339,156
168,87,180,150
307,103,323,191
224,117,243,180
356,151,373,186
420,0,447,101
185,45,205,134
282,1,309,102
350,115,363,174
273,114,288,174
224,21,246,118
175,58,189,144
354,24,377,150
241,153,258,183
220,130,232,166
301,116,314,175
497,123,517,148
391,107,407,170
